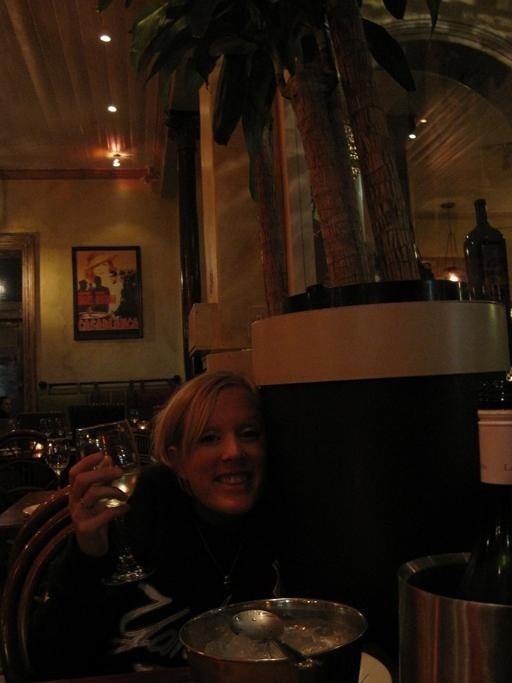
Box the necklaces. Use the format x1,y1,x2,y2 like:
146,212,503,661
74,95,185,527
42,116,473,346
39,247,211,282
190,509,248,592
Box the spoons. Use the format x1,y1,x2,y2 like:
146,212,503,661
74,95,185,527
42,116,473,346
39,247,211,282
229,609,316,671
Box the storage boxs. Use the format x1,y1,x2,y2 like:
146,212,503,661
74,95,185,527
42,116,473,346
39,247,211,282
187,304,269,359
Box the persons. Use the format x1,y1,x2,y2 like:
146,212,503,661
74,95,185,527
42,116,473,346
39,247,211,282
77,252,139,319
66,367,305,676
2,395,15,421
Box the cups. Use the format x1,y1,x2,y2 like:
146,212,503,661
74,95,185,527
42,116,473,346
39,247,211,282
478,400,512,486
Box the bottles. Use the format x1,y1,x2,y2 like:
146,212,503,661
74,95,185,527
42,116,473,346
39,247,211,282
464,200,509,306
482,494,510,605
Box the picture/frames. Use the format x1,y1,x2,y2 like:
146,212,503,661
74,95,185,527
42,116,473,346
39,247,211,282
71,246,143,340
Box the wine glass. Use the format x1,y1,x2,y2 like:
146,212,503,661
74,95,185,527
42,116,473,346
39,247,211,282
77,422,157,589
47,437,69,492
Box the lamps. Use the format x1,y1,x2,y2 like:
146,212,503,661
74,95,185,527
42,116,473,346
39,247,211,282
436,203,465,283
